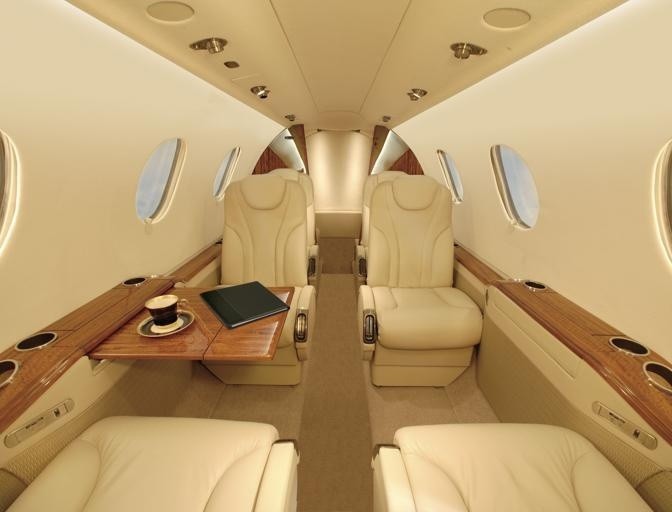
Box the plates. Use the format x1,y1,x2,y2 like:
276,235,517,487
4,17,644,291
136,310,195,336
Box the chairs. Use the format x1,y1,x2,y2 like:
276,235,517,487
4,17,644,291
201,174,317,385
267,167,319,296
356,174,483,388
354,170,413,298
368,422,654,512
0,415,301,512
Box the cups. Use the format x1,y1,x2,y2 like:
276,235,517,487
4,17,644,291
145,293,190,329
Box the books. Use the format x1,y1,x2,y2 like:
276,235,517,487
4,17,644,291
198,281,291,330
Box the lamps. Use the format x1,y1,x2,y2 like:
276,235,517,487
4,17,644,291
287,115,294,121
251,85,267,98
450,42,487,59
193,38,224,54
407,89,427,101
